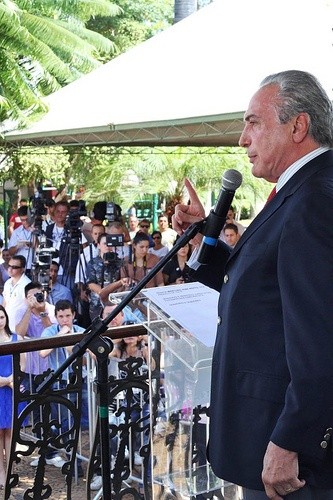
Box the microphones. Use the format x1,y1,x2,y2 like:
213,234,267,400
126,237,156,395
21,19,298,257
197,168,242,265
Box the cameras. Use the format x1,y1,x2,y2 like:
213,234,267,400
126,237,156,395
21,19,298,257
34,293,44,302
130,284,135,291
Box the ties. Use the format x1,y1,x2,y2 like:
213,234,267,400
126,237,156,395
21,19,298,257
265,186,276,207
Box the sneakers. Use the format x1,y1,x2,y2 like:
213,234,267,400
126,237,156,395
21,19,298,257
134,451,142,465
46,456,67,467
30,455,41,466
90,475,103,490
111,471,133,483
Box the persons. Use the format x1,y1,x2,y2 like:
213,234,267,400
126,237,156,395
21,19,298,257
0,306,25,490
0,198,192,490
219,205,245,248
171,70,333,500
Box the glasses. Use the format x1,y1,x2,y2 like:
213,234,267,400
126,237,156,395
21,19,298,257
140,225,149,228
153,236,161,239
8,264,23,269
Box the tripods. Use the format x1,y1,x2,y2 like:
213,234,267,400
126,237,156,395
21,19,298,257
57,236,87,292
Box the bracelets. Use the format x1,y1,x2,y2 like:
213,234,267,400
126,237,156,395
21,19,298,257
145,343,148,346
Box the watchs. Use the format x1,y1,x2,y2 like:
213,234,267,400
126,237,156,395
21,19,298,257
40,312,48,316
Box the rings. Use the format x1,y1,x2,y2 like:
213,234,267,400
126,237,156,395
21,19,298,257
286,486,292,492
